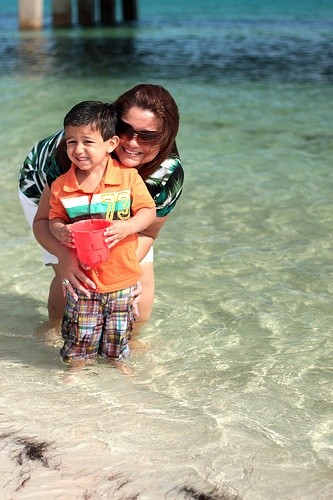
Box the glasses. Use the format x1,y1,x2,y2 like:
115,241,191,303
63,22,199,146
114,119,163,143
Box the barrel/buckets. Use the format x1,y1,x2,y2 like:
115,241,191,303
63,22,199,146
69,219,110,268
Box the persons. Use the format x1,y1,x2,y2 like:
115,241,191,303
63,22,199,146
18,83,184,348
46,99,145,377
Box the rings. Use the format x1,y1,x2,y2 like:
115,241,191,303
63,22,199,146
62,277,69,287
